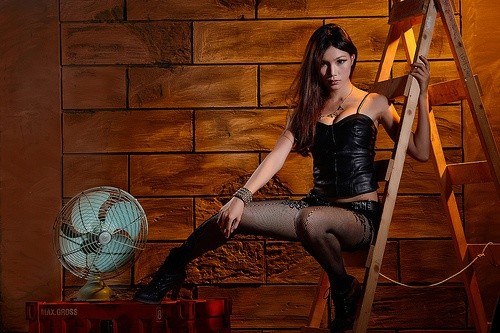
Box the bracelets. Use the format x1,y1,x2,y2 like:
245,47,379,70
233,188,253,207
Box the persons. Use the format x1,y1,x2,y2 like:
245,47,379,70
132,22,432,333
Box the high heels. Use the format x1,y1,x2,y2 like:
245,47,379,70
134,257,187,305
322,275,362,333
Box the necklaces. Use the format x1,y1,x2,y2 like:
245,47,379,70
321,88,353,119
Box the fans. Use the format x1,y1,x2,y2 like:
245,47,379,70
52,186,149,302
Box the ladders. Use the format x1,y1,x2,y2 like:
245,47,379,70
301,0,500,333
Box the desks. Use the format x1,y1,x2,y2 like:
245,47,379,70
26,296,232,333
